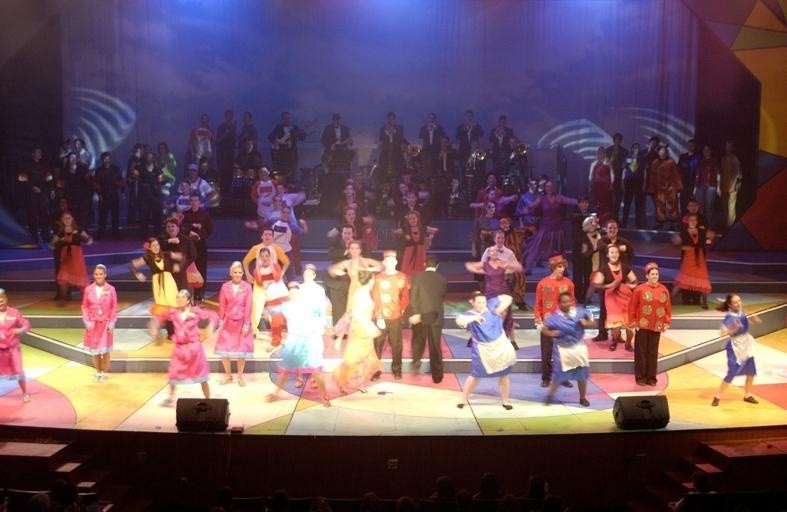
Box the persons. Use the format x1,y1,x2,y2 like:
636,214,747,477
49,197,75,301
360,492,382,512
395,191,432,226
321,114,352,175
161,218,200,307
128,237,182,308
81,264,118,381
388,182,430,207
50,211,94,307
263,194,296,228
432,137,456,220
525,475,546,498
352,170,367,199
266,489,290,512
454,290,517,411
591,219,634,343
128,143,142,222
392,212,439,290
518,181,542,225
239,112,258,138
539,292,597,407
50,479,66,496
140,153,164,239
496,136,528,192
580,215,607,307
267,111,298,187
264,280,330,408
259,183,307,208
455,489,473,512
678,199,708,305
606,133,629,226
400,168,419,188
722,139,742,227
69,166,94,242
189,113,216,176
469,185,518,216
523,180,580,273
641,137,666,227
244,204,308,283
212,260,254,388
198,156,217,180
179,192,215,301
235,138,263,172
318,281,334,328
646,143,685,232
148,289,219,407
326,207,376,238
22,146,56,243
339,179,365,210
216,109,239,196
542,493,566,512
0,288,31,404
166,180,193,212
418,111,446,179
490,115,514,176
464,246,523,351
379,111,405,176
442,176,466,218
475,173,505,218
480,229,519,266
627,262,673,386
591,244,639,350
252,247,289,354
55,484,78,512
26,492,52,512
178,163,215,205
571,194,593,304
327,240,385,396
472,473,509,500
92,152,124,230
460,139,485,218
395,497,416,511
324,224,368,339
155,143,177,200
496,495,521,511
587,147,616,222
621,142,650,228
298,262,324,313
667,471,717,512
165,211,201,241
534,253,576,388
670,212,716,309
695,145,723,227
250,168,287,204
408,254,447,383
208,487,234,512
429,476,454,501
65,153,87,175
369,249,410,382
471,199,502,294
241,226,291,339
310,495,331,512
712,293,763,408
456,110,484,154
142,144,153,166
479,217,536,312
73,139,90,165
677,140,702,216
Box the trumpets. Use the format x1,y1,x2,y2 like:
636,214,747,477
518,142,529,157
475,150,486,161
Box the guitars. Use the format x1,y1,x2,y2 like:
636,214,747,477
335,127,368,147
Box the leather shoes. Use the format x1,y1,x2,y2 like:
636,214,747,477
592,335,608,341
411,361,422,369
712,398,719,405
609,343,618,351
542,380,549,387
579,398,588,405
467,339,472,347
692,301,701,305
618,336,625,343
433,377,441,383
744,396,758,404
511,341,519,350
562,382,574,387
516,303,528,311
638,381,647,386
624,345,634,351
395,373,400,378
371,371,382,381
239,378,244,386
504,404,514,409
536,261,545,267
525,270,532,275
218,375,233,385
682,300,691,305
458,403,463,408
650,382,656,386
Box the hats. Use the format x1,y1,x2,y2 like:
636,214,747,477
305,264,316,271
384,250,397,257
549,255,564,263
188,164,197,170
646,263,660,272
257,167,270,178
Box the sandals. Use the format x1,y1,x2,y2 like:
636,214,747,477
322,398,330,407
266,393,276,402
294,377,303,387
310,378,317,388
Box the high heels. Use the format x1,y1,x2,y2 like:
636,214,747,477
357,387,368,394
340,388,348,396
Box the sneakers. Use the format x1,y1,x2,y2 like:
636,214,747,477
22,394,31,402
93,372,101,382
99,373,109,382
265,346,275,352
159,398,173,407
